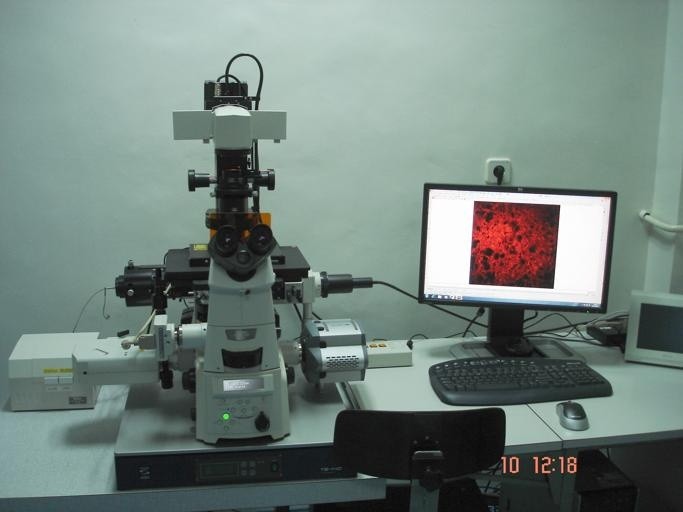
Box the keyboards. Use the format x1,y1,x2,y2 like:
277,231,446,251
428,355,614,406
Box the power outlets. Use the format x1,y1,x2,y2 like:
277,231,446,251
482,153,515,187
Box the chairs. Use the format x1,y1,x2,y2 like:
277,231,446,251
333,408,507,512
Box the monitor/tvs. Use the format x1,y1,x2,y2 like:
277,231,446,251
418,182,618,360
625,289,682,370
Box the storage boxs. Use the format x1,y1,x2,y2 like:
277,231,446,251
6,331,100,412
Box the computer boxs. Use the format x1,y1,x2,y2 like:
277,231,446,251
574,450,641,512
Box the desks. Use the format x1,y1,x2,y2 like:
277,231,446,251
341,321,681,512
1,336,385,512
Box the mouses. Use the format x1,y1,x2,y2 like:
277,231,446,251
555,398,590,431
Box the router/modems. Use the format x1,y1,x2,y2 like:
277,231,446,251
587,321,624,344
